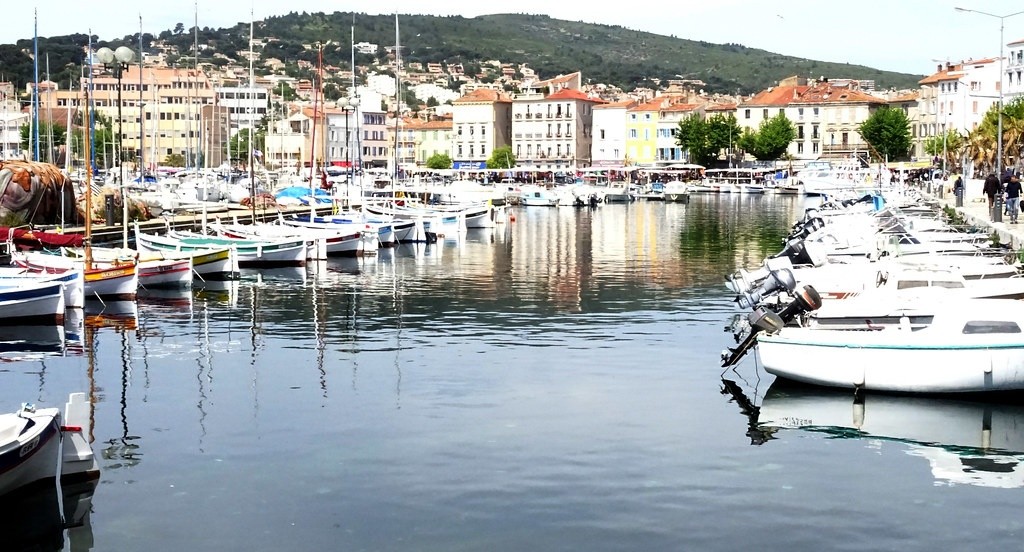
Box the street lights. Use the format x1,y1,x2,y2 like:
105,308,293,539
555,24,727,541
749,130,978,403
934,59,967,198
336,97,360,198
727,110,734,168
97,46,136,225
955,7,1023,182
921,85,953,180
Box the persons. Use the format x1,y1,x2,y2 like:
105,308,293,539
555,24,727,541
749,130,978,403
934,154,938,161
983,173,1024,224
953,177,963,196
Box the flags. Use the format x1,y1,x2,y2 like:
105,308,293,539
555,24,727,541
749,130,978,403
254,149,264,157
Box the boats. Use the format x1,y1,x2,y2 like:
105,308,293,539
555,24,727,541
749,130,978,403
721,191,1024,392
0,405,64,501
0,5,508,323
721,377,1024,490
795,134,895,194
503,178,720,205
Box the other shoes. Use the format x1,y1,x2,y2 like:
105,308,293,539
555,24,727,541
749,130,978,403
1010,222,1013,224
1015,221,1017,224
